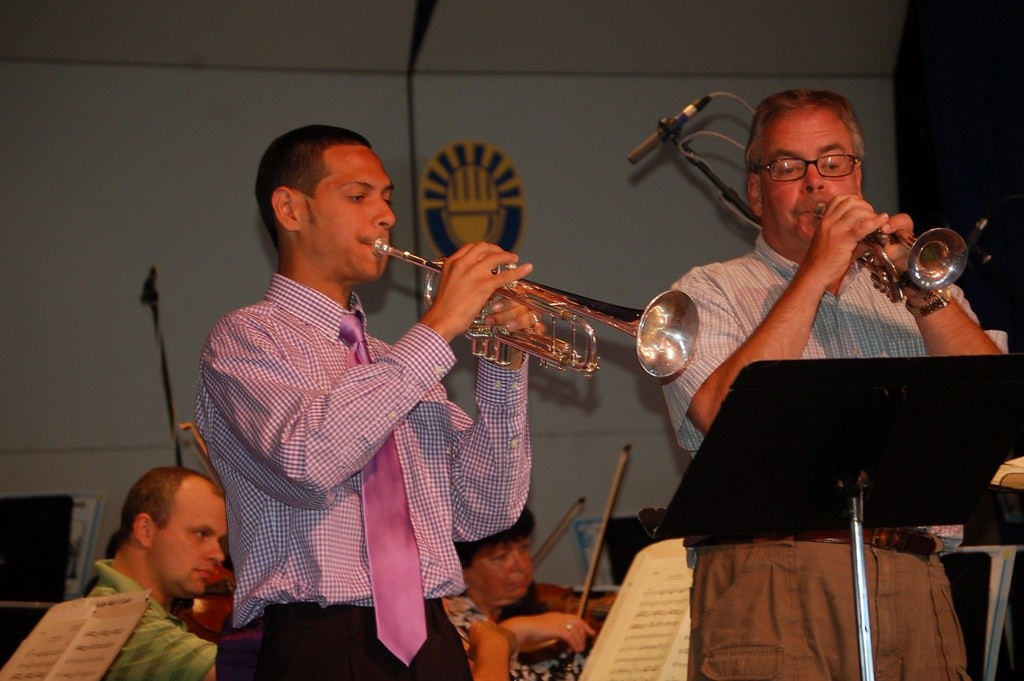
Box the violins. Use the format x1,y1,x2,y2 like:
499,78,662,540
170,559,238,642
488,580,618,665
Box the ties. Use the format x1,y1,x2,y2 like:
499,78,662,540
339,314,428,668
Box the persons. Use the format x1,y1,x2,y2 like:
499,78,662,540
649,86,1009,681
196,123,546,681
77,463,232,681
438,502,597,681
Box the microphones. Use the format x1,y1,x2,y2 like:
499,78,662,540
626,96,709,166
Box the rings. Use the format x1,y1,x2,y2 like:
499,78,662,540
529,309,539,325
563,624,574,630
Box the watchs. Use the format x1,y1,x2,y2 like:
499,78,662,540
905,286,952,317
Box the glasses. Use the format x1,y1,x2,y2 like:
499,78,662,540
755,153,861,183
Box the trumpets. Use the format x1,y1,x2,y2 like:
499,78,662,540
815,200,968,305
373,236,701,379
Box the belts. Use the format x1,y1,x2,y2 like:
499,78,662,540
762,524,945,561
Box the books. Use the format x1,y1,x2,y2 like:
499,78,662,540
581,536,702,681
